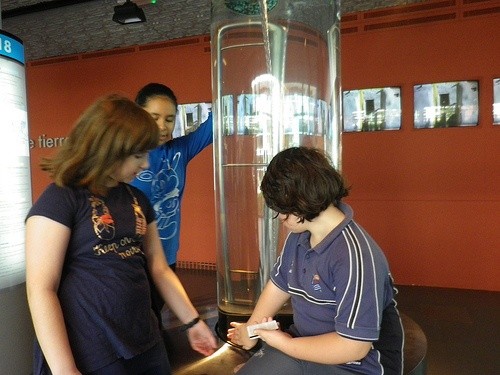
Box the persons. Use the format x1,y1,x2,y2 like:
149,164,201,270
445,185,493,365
227,145,405,375
24,93,218,375
131,83,213,329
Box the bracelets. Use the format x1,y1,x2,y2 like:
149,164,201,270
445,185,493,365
180,317,201,332
